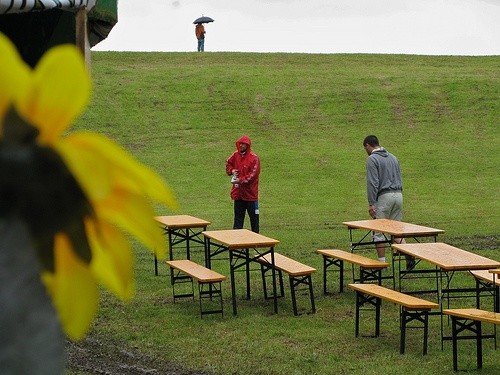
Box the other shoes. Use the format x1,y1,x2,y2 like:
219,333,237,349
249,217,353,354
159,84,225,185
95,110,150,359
405,254,416,270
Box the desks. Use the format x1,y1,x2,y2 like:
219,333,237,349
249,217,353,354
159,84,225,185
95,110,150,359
201,229,280,316
342,218,445,305
151,215,211,283
390,242,500,324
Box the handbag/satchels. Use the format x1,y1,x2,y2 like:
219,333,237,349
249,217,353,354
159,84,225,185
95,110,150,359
200,34,204,39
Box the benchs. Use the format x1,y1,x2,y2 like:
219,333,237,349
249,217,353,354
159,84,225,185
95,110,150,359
254,252,317,317
165,260,227,320
317,249,389,306
471,269,500,311
441,308,500,372
348,283,439,356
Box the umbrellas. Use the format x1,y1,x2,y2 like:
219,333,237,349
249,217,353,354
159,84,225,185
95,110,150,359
193,16,214,24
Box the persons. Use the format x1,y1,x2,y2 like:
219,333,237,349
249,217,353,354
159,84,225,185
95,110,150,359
363,134,406,264
196,23,206,52
227,136,260,234
